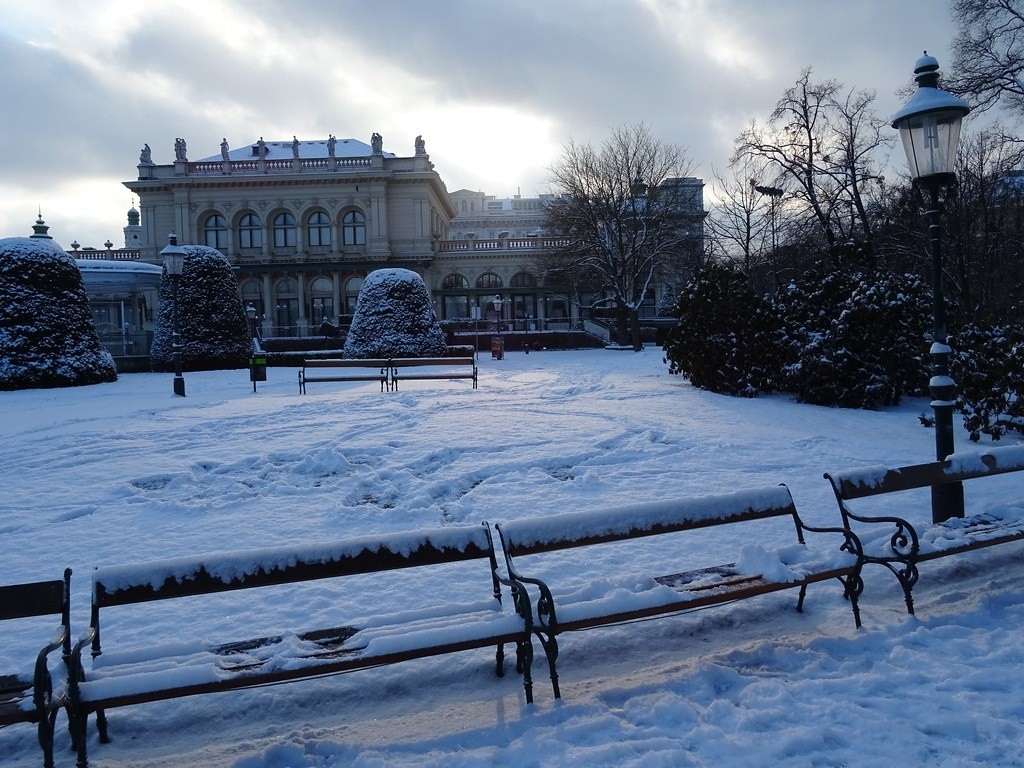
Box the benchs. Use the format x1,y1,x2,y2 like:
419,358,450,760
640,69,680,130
298,358,389,395
495,482,867,703
822,443,1023,618
70,519,533,767
0,567,75,767
390,356,478,391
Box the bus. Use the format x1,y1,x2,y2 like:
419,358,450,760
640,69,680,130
483,298,569,331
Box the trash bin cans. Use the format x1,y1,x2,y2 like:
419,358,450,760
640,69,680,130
530,324,535,330
507,323,514,331
249,353,266,381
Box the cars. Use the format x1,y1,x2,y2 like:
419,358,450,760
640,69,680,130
98,336,135,354
95,322,124,335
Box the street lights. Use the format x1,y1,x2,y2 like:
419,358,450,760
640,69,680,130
160,232,187,397
125,322,130,355
95,309,105,323
246,302,256,392
493,296,503,337
889,51,971,524
524,311,529,354
